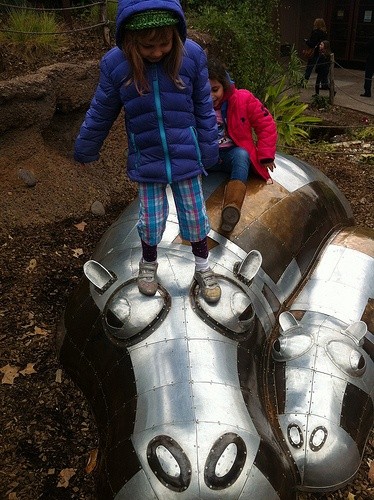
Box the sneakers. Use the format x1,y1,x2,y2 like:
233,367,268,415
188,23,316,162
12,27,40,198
194,267,221,303
138,257,159,296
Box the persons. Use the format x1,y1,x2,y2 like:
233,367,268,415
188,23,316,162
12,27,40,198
206,56,279,233
71,0,222,303
360,40,374,97
298,18,338,98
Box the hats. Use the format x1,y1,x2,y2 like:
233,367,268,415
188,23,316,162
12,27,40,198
122,10,181,29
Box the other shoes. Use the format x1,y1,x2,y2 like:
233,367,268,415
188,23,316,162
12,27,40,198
360,93,371,97
312,94,319,97
334,91,337,96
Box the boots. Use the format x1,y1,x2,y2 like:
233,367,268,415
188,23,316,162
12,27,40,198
220,178,247,233
